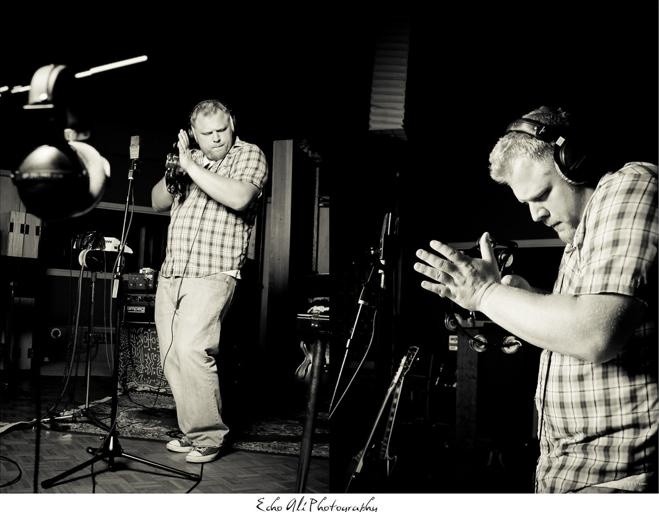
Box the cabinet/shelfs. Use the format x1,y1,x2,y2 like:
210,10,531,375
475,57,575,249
0,168,265,379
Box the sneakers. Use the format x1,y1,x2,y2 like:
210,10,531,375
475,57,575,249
166,437,193,453
185,447,222,462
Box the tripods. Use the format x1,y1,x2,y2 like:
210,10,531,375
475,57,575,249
46,269,118,435
42,161,201,490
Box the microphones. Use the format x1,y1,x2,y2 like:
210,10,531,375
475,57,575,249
130,135,140,160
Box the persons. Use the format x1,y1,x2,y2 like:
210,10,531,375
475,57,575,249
148,98,273,464
412,103,658,491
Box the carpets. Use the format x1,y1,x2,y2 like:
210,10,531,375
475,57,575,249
35,390,365,458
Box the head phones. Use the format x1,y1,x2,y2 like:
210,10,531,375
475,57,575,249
506,117,598,184
187,98,239,146
